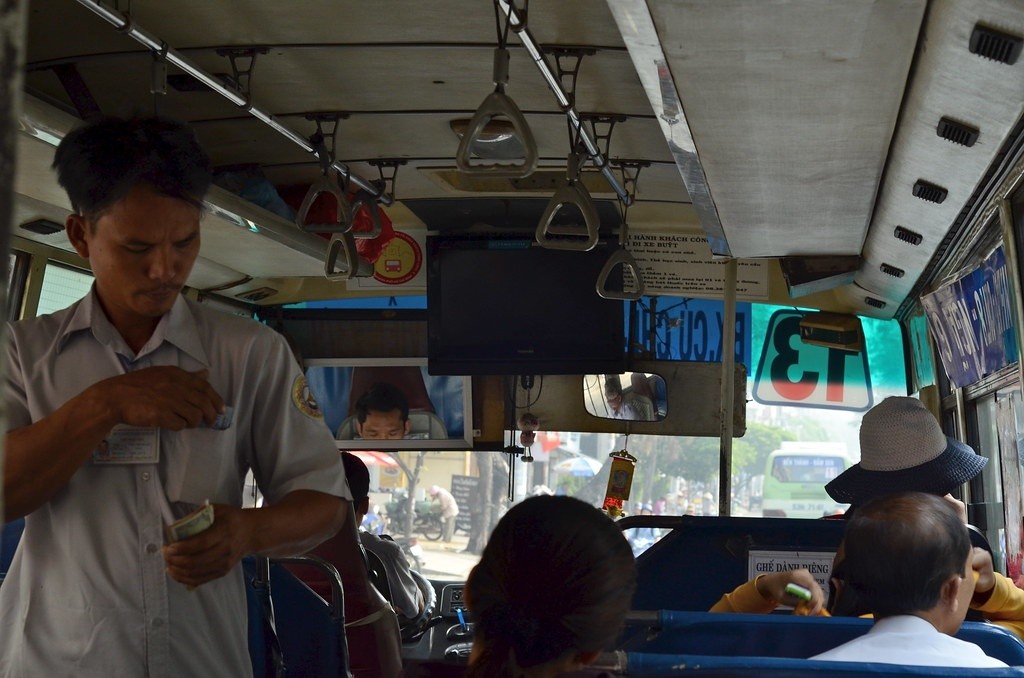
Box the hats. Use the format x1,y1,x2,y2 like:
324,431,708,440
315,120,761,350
825,396,987,504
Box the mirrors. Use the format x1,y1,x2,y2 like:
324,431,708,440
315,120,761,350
301,358,473,449
584,372,668,421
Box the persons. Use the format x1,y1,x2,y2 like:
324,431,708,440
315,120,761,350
826,391,992,536
802,488,1012,669
348,380,425,440
326,445,430,678
711,500,1023,647
603,379,642,421
436,492,638,677
0,111,354,678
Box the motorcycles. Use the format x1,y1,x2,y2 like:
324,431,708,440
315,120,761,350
358,505,429,576
385,493,443,541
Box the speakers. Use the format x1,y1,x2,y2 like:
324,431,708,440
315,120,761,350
799,311,865,352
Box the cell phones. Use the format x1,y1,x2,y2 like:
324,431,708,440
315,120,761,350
785,583,813,603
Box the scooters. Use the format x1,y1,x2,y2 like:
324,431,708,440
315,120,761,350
624,536,661,559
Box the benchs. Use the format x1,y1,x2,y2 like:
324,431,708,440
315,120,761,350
620,613,1023,667
594,653,1022,678
0,556,351,678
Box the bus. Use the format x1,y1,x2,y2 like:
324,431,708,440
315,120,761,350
759,441,854,520
